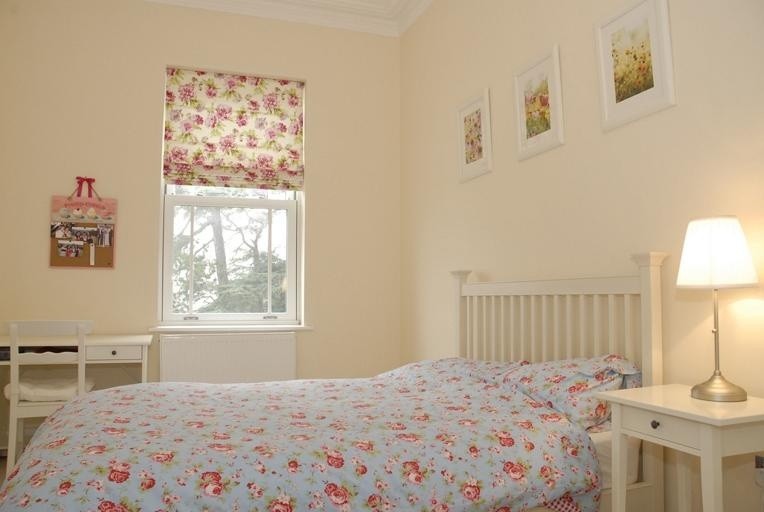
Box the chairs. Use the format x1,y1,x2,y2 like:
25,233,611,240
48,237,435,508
4,320,94,477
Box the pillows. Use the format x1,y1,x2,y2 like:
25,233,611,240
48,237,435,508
499,354,640,429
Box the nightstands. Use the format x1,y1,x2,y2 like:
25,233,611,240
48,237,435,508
598,384,764,512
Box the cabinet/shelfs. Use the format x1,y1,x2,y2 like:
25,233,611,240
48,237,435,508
1,334,154,384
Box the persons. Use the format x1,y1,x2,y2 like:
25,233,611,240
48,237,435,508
55,225,113,257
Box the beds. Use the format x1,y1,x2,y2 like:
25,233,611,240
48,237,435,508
1,250,667,512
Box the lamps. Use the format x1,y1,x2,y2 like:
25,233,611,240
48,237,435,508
675,215,760,402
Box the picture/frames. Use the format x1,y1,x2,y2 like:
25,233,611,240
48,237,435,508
512,42,564,161
597,0,678,132
454,87,496,183
